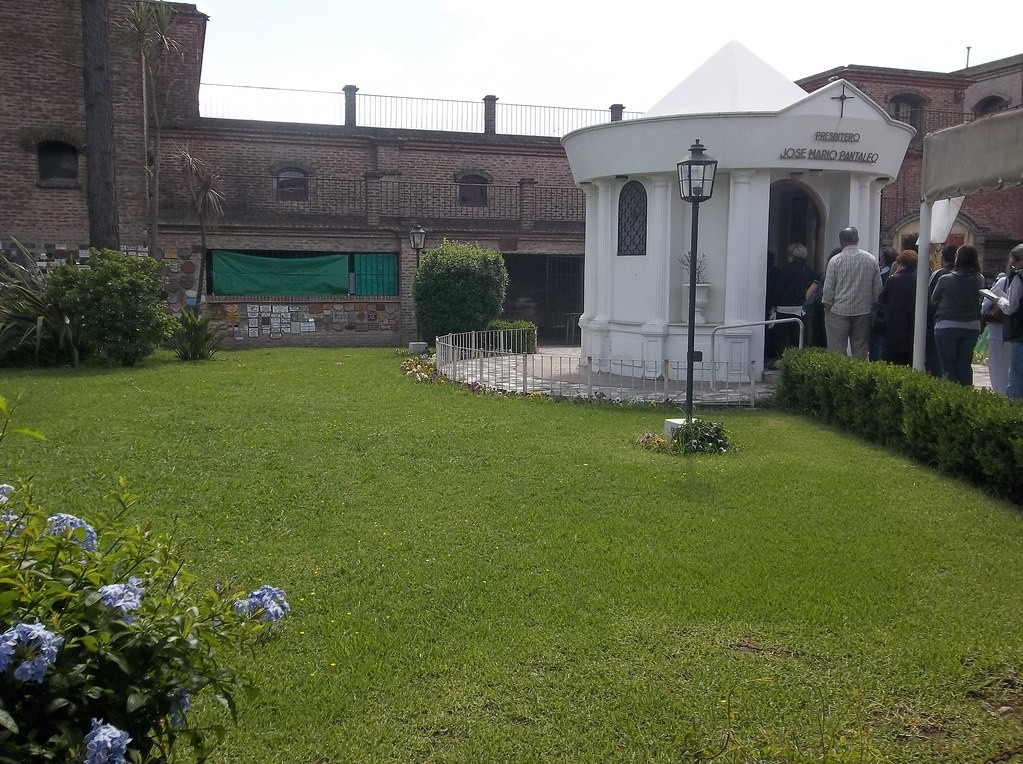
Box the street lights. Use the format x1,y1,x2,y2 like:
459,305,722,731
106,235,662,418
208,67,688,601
674,136,720,426
408,222,427,343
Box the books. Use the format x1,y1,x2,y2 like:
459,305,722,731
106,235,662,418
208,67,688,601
977,283,1008,302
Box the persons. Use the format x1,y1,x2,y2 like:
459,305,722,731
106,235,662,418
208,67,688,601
800,247,843,350
876,247,899,281
931,243,986,386
882,249,931,371
980,256,1017,399
994,244,1023,400
805,270,826,301
771,242,817,361
927,243,958,297
821,225,884,363
